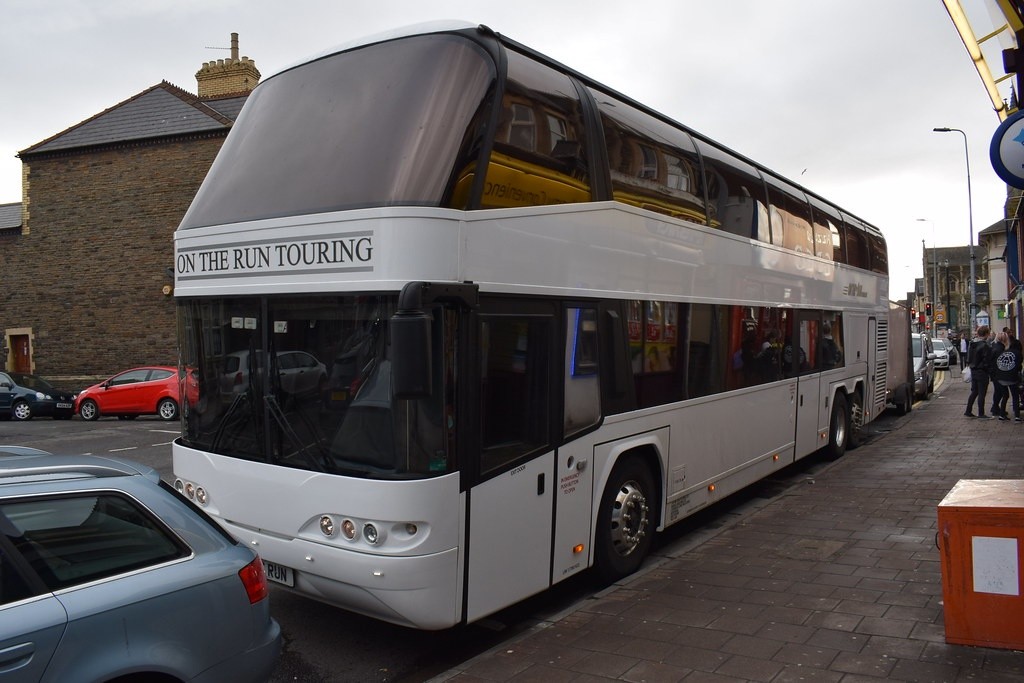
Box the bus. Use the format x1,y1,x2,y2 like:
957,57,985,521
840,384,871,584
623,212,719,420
170,18,889,637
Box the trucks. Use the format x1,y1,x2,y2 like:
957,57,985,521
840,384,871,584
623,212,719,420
888,299,915,414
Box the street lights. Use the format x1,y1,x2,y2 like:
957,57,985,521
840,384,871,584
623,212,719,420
934,127,977,337
916,217,937,310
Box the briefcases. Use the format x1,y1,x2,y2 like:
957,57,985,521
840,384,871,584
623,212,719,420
962,366,971,383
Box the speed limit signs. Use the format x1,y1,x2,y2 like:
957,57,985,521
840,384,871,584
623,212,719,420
935,310,946,322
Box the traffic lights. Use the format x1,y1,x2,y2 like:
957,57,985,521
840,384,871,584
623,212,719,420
926,302,933,316
911,310,916,318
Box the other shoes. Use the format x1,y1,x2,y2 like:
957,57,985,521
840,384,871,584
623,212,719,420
1019,404,1024,410
990,407,1008,415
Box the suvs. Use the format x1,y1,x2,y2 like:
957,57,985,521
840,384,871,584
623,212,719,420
911,330,937,399
931,337,958,364
218,348,327,408
1,441,280,681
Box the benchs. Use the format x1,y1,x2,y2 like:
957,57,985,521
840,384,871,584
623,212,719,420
70,511,176,571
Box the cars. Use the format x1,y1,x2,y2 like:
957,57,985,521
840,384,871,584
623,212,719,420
178,365,199,417
931,340,949,370
1,371,75,423
75,365,180,421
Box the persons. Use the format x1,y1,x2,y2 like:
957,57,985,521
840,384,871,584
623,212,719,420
763,333,782,375
955,326,1024,421
819,323,837,368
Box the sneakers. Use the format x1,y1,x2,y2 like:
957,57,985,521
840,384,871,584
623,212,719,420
978,415,995,420
999,416,1010,422
964,412,978,419
992,413,999,419
1015,417,1024,423
